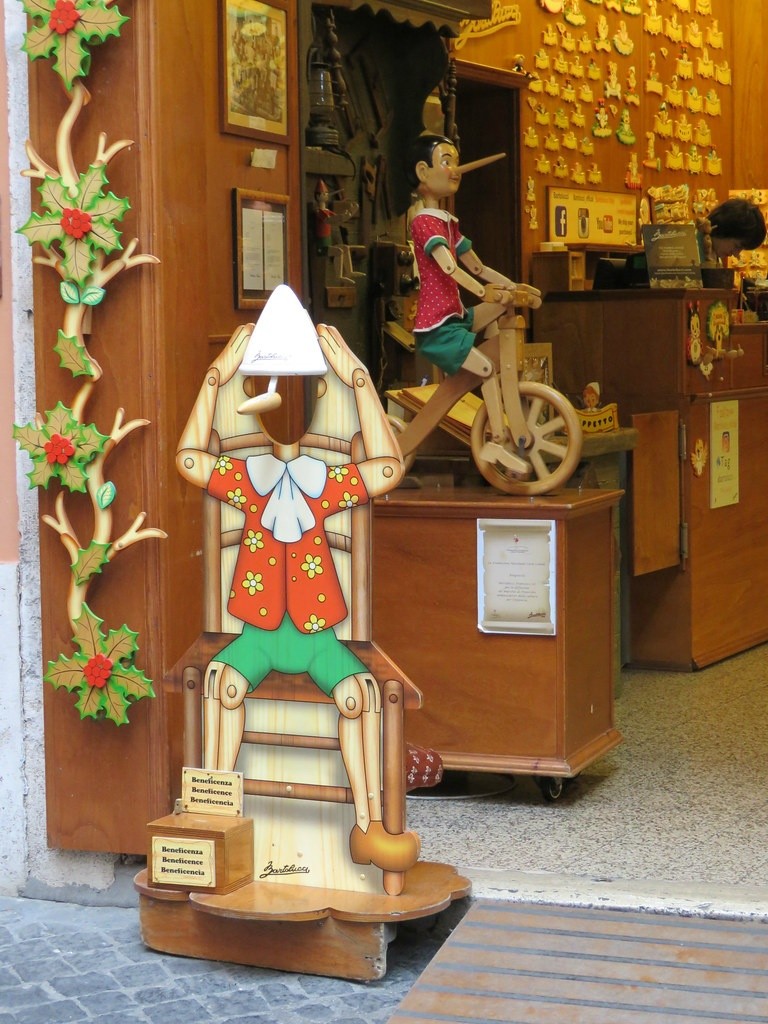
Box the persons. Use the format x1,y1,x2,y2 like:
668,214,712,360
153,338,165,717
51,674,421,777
687,196,766,269
408,133,543,475
174,285,419,874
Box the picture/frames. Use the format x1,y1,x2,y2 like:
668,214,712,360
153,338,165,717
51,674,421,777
218,0,292,146
233,187,291,311
546,184,638,247
520,341,556,425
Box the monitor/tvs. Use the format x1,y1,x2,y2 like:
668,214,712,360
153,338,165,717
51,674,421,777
592,258,628,290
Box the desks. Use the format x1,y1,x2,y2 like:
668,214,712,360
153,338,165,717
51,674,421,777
411,426,642,698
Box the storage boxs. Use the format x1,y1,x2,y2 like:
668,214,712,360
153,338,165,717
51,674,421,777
701,268,735,289
533,252,586,291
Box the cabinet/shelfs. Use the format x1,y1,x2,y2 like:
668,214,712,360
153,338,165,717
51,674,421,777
206,488,627,801
